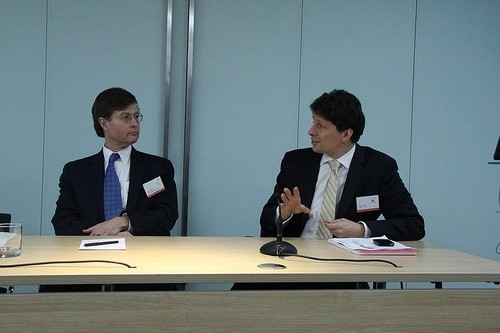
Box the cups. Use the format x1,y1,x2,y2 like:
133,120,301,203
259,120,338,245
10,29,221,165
0,223,23,258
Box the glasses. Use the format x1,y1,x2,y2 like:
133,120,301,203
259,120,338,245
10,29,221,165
110,113,143,124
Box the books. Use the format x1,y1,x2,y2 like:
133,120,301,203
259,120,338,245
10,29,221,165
328,234,418,257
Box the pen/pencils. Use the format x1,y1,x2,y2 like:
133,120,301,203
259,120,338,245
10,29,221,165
84,240,119,247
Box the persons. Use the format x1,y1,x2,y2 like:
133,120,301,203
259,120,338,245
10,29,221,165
260,90,426,241
51,87,180,236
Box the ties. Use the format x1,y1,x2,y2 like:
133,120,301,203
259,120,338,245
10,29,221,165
317,160,342,239
104,153,123,220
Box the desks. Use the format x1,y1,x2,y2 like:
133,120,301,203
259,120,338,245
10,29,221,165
0,236,500,332
